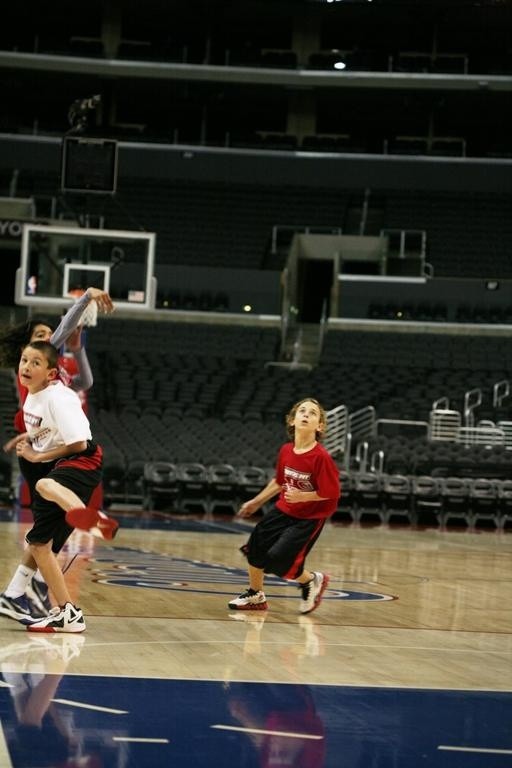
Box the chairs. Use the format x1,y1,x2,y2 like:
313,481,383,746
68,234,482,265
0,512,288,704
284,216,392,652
0,165,512,530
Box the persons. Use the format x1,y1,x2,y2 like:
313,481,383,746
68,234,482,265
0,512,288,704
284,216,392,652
4,339,124,635
225,396,342,616
1,284,118,627
1,634,90,768
222,610,329,768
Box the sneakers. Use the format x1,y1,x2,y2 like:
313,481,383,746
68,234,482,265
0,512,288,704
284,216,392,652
0,578,86,633
66,505,120,540
229,590,266,610
301,571,328,614
